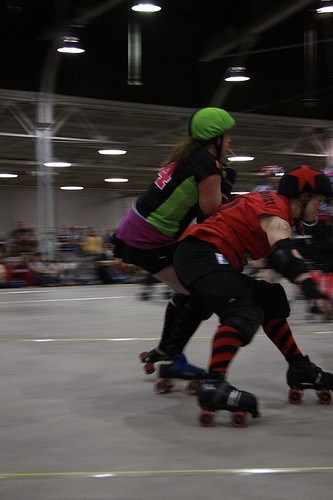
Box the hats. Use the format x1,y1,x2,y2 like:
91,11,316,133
276,164,332,200
187,107,236,143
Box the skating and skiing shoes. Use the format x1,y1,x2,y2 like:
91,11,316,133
283,353,333,407
195,375,260,429
139,333,183,375
152,352,207,397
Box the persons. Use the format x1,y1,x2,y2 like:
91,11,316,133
242,165,333,322
174,165,333,427
110,107,236,395
0,222,174,301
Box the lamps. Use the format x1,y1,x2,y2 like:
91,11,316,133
55,21,89,55
315,0,333,14
224,60,252,82
131,0,163,14
